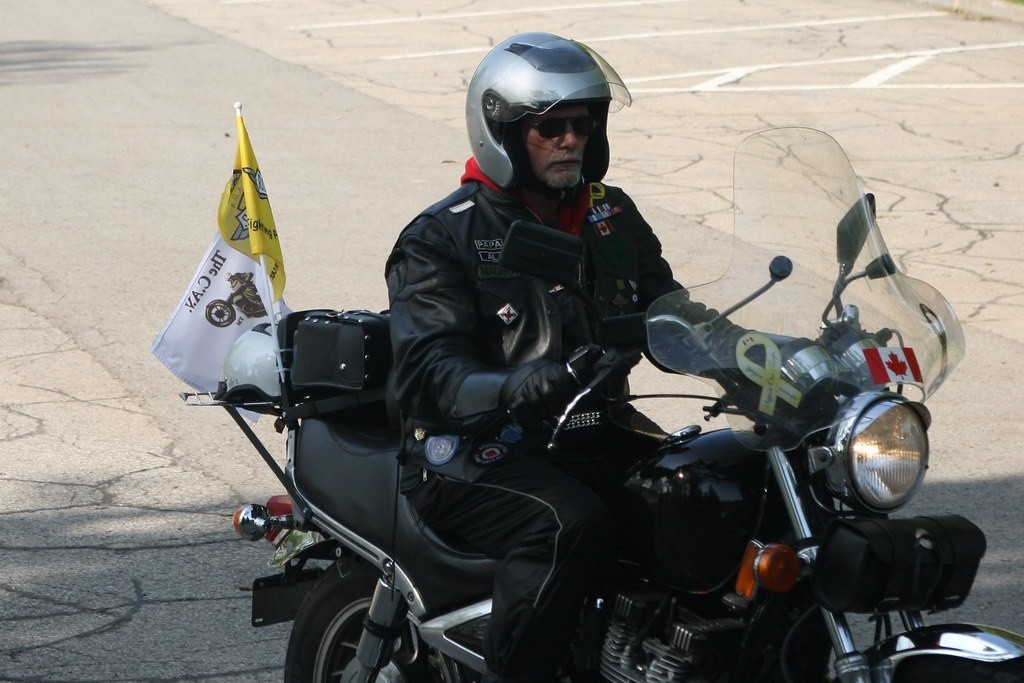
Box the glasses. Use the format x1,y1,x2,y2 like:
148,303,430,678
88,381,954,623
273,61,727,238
522,115,595,138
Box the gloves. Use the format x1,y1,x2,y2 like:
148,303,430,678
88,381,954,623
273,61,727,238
451,358,587,431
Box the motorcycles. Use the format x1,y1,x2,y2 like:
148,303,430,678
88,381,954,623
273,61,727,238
176,126,1024,683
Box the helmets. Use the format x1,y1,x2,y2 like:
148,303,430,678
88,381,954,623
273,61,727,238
465,32,612,192
212,323,283,401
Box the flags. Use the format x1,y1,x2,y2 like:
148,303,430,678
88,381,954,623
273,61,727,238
154,116,285,424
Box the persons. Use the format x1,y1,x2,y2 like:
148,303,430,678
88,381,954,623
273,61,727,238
385,34,829,683
